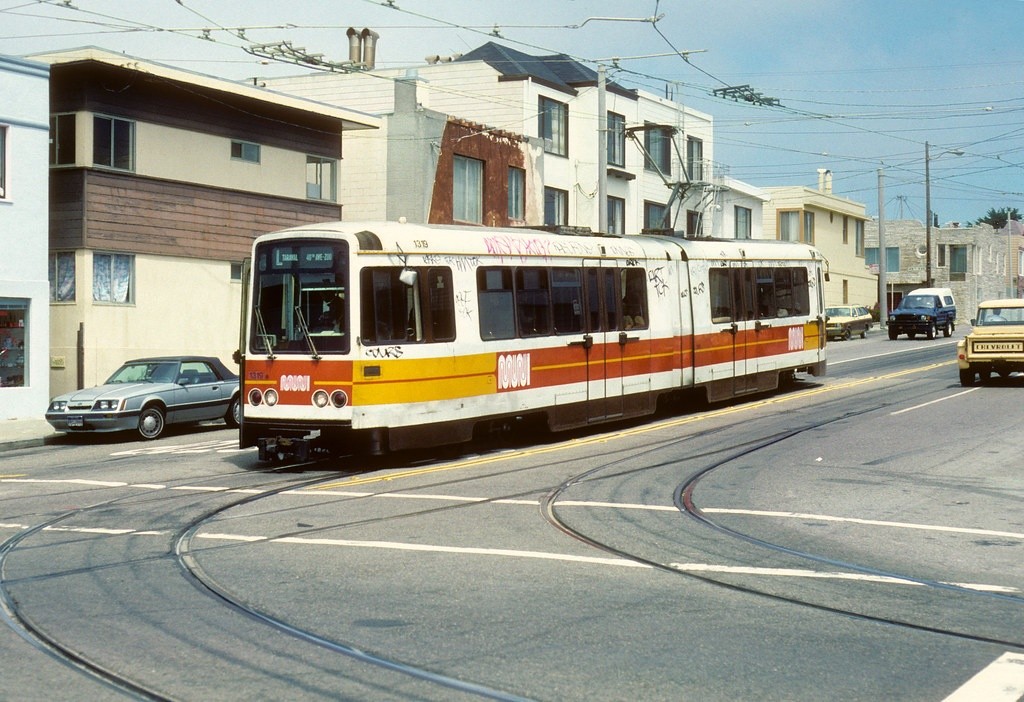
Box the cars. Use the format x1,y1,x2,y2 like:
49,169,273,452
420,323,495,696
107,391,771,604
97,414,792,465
957,298,1024,387
44,355,239,440
825,305,874,341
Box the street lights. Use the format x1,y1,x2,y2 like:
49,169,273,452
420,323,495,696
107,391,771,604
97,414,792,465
924,140,965,287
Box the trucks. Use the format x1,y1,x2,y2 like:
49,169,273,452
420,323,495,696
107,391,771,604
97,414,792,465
885,287,958,341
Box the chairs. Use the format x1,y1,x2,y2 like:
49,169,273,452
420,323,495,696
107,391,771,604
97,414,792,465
180,369,201,383
199,373,212,381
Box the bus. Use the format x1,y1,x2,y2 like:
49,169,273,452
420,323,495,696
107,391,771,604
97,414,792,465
229,215,832,472
